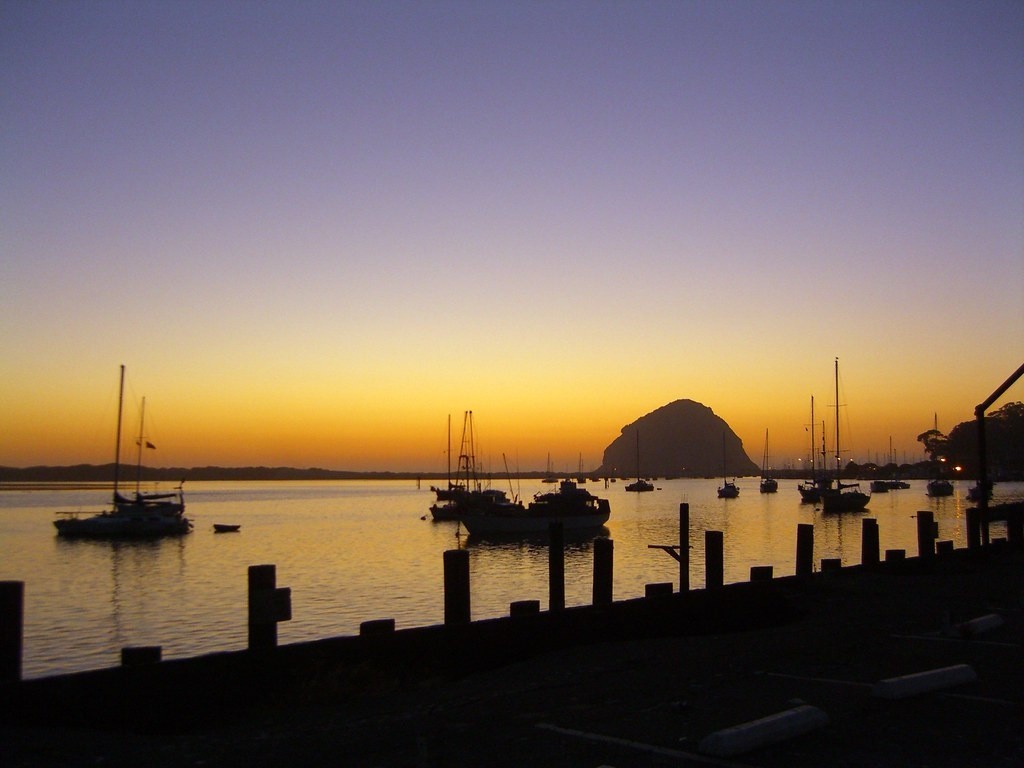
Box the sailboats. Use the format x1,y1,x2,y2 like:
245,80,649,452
427,411,615,541
760,428,781,495
797,356,995,513
54,364,194,541
717,425,741,499
624,429,655,494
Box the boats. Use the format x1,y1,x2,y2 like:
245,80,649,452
212,520,242,532
